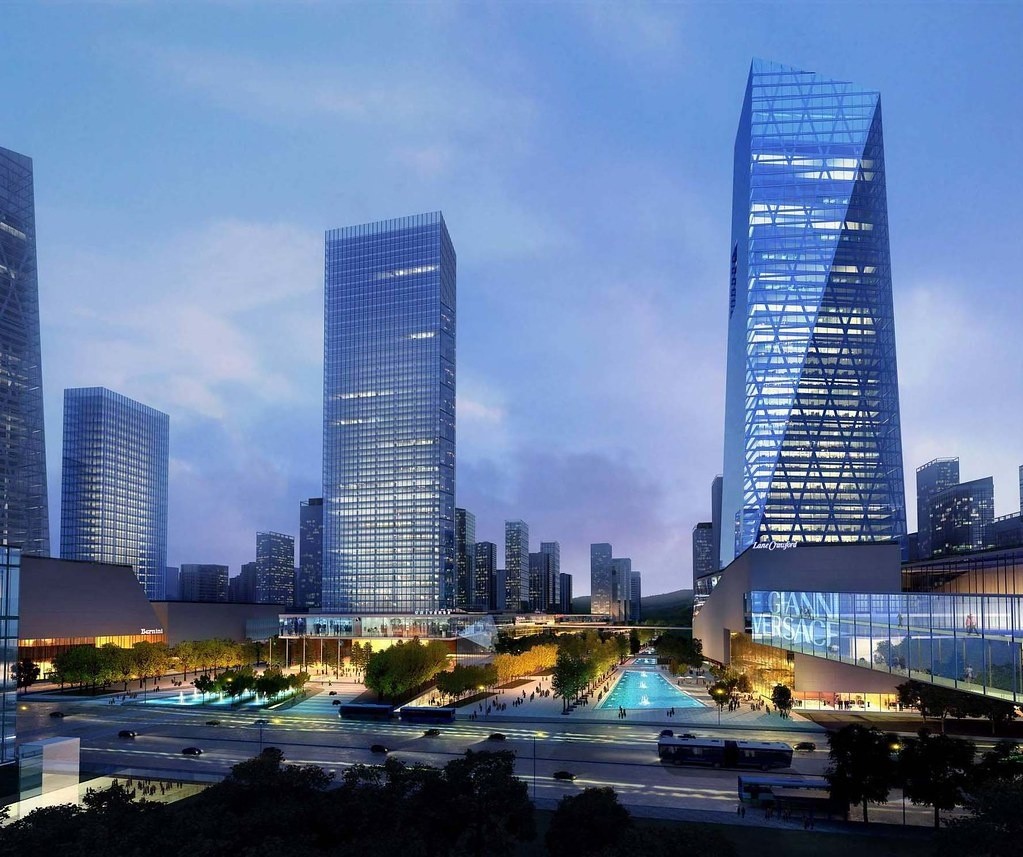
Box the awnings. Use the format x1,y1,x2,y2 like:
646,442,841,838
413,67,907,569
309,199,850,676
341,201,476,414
17,554,165,642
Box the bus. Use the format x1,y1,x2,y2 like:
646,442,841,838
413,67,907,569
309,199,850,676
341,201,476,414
738,777,852,820
659,737,793,770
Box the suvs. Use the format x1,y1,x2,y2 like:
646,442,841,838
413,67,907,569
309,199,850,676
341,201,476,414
794,742,817,751
423,729,440,736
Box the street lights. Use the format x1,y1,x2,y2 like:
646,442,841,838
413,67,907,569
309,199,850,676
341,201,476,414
479,686,487,719
532,732,544,801
891,745,906,827
132,675,147,706
716,688,723,726
227,678,233,712
255,720,269,755
16,706,27,759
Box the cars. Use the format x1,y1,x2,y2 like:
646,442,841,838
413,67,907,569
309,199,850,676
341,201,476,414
181,747,203,755
370,745,389,752
48,712,65,719
205,720,220,727
119,731,137,739
489,734,506,740
554,771,577,781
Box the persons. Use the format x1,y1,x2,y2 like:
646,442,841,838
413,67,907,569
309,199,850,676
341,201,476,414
824,699,904,711
897,612,903,628
572,663,615,706
86,778,182,794
428,676,550,720
619,705,626,719
964,664,972,689
736,805,815,830
317,665,365,687
966,613,980,636
892,654,906,674
109,672,217,705
729,693,802,720
678,669,708,690
828,643,838,658
667,707,675,717
800,606,812,619
